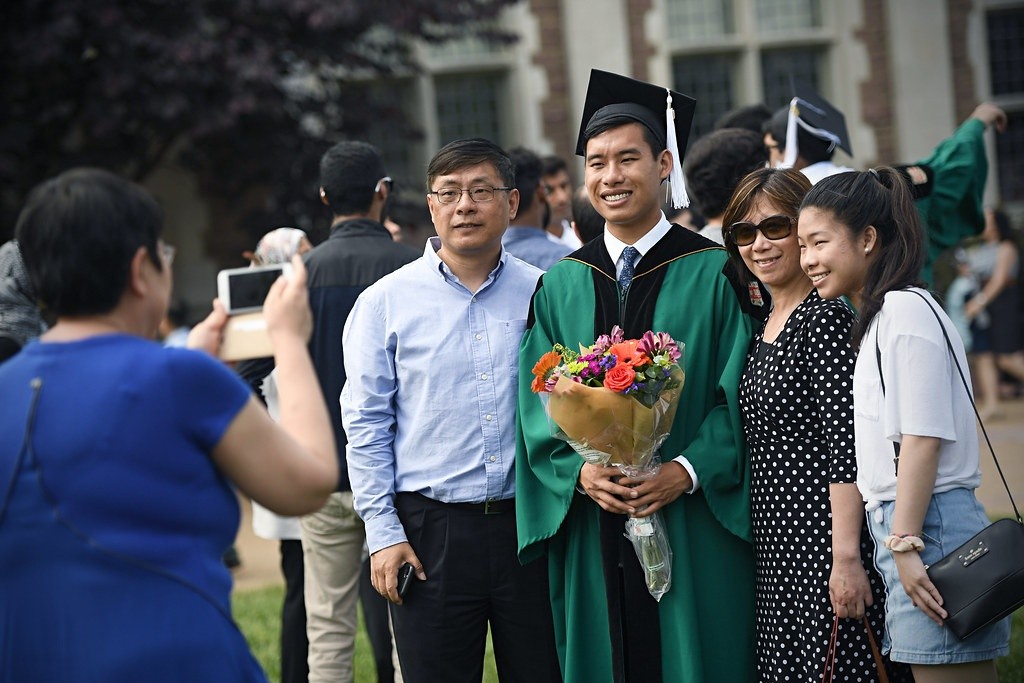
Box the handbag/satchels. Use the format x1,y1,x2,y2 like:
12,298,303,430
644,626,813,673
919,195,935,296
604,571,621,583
927,518,1024,643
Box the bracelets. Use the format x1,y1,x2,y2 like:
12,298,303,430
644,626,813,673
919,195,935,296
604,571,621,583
884,533,925,553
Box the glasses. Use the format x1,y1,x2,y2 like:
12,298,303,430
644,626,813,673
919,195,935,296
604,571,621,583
143,247,178,265
724,215,796,246
430,185,510,203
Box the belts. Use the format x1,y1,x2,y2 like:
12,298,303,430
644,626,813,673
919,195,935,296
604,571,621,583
448,501,516,514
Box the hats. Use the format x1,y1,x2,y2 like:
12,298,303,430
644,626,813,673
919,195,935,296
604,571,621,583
771,85,853,168
577,68,698,210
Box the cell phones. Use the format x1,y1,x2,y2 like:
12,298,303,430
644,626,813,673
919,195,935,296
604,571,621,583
396,563,414,597
217,263,291,315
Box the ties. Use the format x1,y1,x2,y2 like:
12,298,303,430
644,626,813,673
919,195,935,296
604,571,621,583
619,246,639,302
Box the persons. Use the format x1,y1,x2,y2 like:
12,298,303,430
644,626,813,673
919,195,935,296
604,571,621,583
244,227,396,683
721,165,913,683
514,69,754,683
232,140,423,683
0,168,339,683
340,137,566,683
796,167,1010,682
1,90,1024,425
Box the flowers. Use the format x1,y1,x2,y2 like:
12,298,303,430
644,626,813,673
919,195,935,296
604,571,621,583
532,324,686,594
